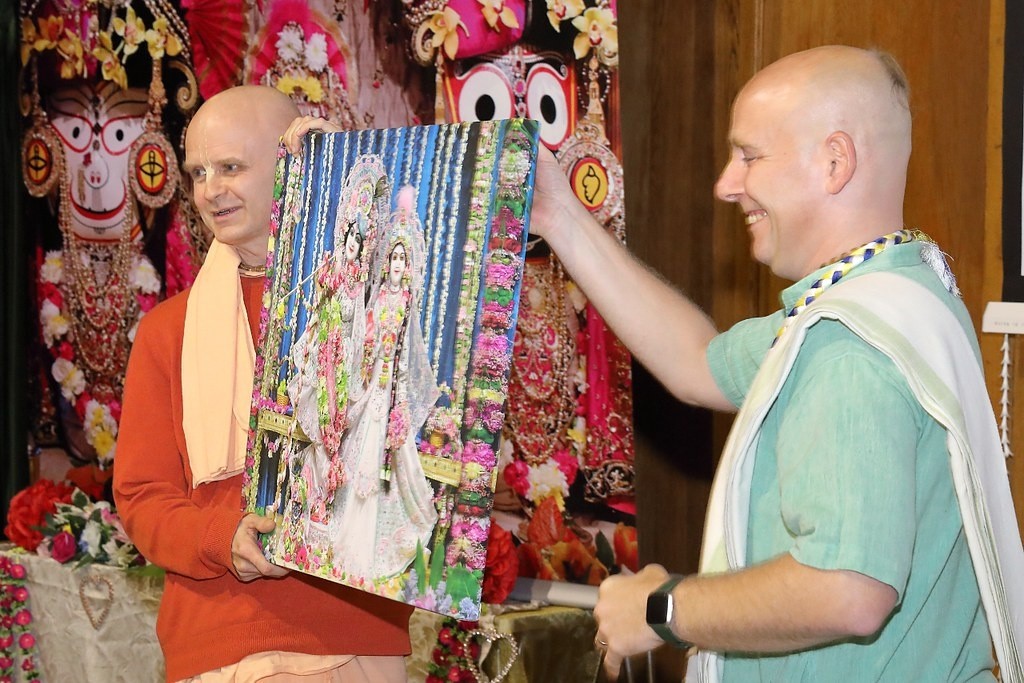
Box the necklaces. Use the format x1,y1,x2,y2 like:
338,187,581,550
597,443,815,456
239,264,266,272
801,241,872,276
768,228,912,349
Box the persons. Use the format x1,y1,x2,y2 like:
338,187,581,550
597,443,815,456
112,86,416,683
521,44,1024,683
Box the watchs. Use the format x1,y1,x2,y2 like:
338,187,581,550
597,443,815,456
646,576,686,646
